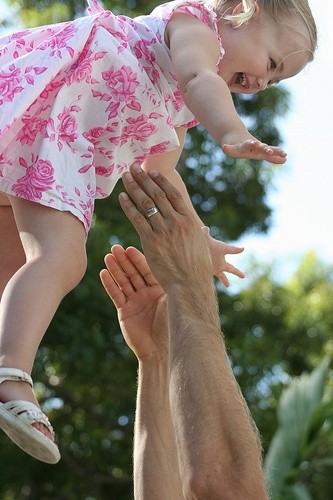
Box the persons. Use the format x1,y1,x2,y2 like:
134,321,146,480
100,162,273,500
0,0,318,465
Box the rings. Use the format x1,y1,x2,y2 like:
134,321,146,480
144,208,158,218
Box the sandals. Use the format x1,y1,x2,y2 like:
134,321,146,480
1,368,61,465
